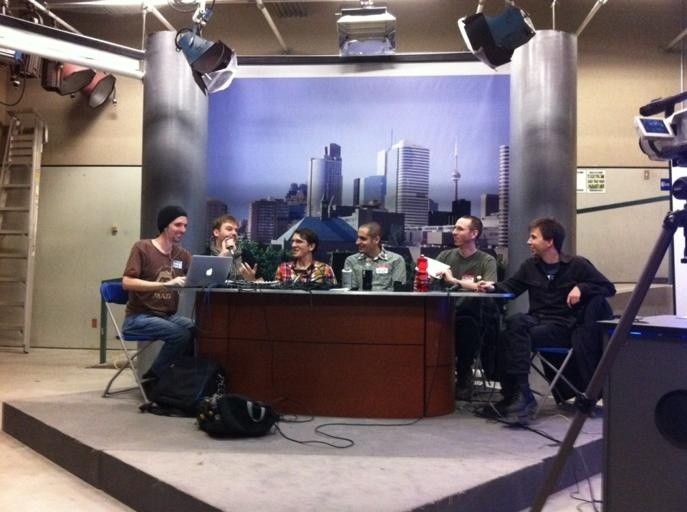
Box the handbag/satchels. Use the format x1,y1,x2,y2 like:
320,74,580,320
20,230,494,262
141,356,227,417
200,391,278,437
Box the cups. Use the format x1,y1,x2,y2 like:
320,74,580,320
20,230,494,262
362,269,372,290
342,269,352,290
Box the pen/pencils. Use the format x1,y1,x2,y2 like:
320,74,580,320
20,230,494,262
229,246,235,256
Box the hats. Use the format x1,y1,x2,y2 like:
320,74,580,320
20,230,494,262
156,206,186,232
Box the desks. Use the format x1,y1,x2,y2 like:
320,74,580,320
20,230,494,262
165,281,514,418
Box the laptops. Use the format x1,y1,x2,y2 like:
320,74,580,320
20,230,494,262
164,254,232,288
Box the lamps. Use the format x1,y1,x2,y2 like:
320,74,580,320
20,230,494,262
457,1,535,71
81,69,117,110
58,62,96,95
174,0,237,97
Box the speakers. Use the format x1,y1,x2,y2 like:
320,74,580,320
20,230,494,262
595,314,687,512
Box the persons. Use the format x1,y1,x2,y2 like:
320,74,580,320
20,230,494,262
202,214,264,281
273,228,337,284
121,205,197,398
476,217,616,416
413,214,498,399
344,222,406,291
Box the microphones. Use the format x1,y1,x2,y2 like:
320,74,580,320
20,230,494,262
224,235,235,256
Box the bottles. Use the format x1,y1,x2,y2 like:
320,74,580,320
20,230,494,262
417,253,428,293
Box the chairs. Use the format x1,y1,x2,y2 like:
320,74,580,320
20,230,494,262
453,275,503,389
100,281,160,404
530,294,600,421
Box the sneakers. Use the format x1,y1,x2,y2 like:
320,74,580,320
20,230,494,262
454,377,538,419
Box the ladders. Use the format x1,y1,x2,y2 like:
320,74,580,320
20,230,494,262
0,108,48,353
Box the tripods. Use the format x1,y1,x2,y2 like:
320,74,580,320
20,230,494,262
531,177,687,512
